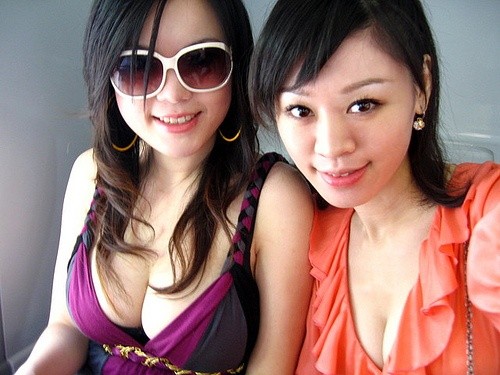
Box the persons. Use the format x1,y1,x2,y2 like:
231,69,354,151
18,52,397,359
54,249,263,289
13,1,314,374
247,0,500,374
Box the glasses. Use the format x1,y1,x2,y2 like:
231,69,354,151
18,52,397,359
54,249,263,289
107,41,236,99
